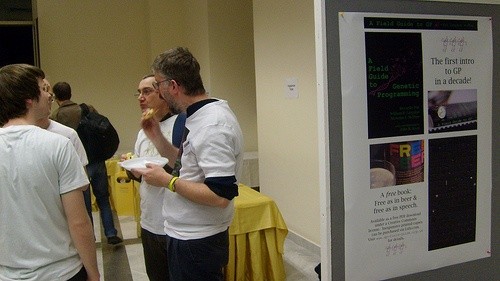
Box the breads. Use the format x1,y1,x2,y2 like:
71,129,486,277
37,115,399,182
125,152,133,160
145,108,156,120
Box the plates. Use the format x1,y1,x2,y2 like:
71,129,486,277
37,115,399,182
121,157,168,170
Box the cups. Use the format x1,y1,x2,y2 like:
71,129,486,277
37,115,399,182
370,159,397,189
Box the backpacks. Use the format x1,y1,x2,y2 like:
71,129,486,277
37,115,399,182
76,103,120,161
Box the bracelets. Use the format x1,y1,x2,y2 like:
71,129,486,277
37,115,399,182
169,176,179,192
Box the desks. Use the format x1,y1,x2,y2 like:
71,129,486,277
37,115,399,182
90,158,289,281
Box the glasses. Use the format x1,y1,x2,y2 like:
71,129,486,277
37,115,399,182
152,79,168,89
134,88,154,97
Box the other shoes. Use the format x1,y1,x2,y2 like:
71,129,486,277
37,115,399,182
107,235,123,245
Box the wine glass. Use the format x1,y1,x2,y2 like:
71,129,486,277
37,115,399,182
133,100,168,127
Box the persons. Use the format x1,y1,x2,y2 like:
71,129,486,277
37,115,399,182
44,75,185,281
0,64,101,281
130,47,243,281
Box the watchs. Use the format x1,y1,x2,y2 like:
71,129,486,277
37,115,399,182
169,177,178,192
